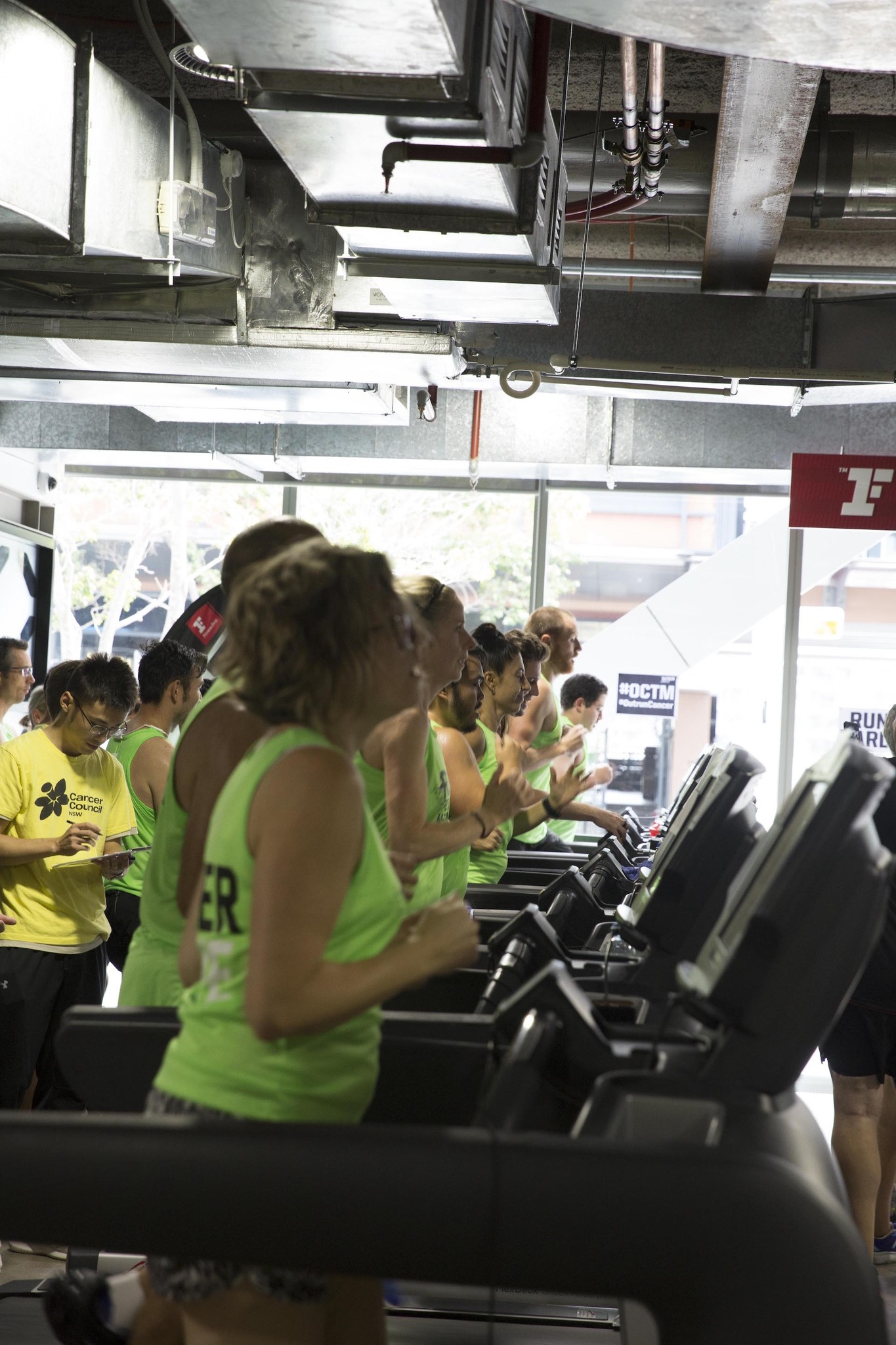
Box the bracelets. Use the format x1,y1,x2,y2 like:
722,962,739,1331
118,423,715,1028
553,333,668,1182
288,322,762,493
542,796,562,819
470,810,485,838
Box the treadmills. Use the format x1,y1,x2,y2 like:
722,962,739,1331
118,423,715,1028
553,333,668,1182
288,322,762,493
0,736,895,1345
374,743,770,1009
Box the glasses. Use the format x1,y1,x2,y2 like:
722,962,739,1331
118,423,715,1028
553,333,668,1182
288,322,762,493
2,667,33,677
76,703,128,736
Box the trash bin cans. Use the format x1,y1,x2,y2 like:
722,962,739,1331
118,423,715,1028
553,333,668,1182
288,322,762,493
642,746,659,801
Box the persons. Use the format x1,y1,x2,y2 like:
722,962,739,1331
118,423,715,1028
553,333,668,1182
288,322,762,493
428,623,595,884
548,673,612,842
507,606,629,853
353,573,531,912
118,516,322,1009
45,536,480,1345
0,635,84,744
107,639,207,973
0,651,138,1110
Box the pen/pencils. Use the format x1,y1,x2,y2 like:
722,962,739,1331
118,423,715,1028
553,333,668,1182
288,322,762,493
66,820,103,836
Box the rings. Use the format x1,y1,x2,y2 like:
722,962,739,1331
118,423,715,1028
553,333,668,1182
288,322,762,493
623,821,627,828
